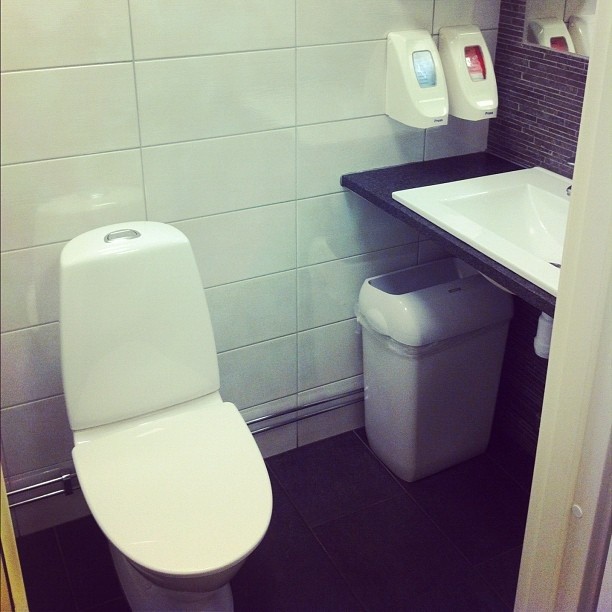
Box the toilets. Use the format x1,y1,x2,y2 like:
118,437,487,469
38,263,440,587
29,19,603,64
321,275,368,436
58,220,274,610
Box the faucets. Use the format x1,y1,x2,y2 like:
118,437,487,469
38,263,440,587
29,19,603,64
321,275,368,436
566,158,575,197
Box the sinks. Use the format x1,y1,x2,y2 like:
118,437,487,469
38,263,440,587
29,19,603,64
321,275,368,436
391,165,572,297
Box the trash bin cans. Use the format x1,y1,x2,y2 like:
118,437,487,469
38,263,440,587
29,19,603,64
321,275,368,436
354,257,517,486
